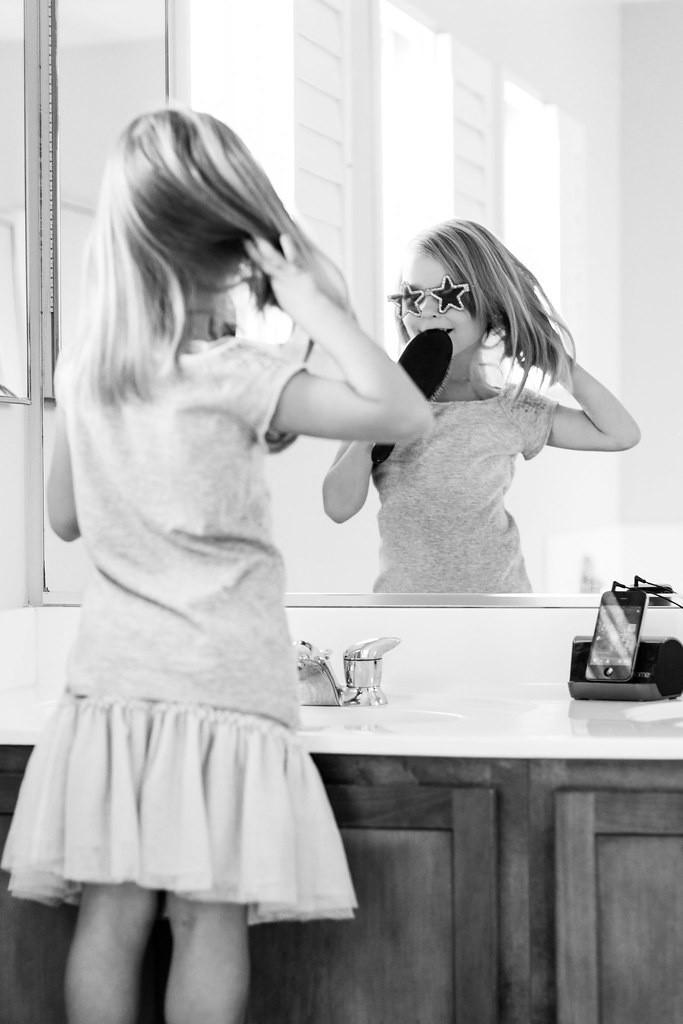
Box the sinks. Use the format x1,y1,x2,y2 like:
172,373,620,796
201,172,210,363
255,703,467,759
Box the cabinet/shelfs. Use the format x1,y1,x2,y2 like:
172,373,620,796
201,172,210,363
530,760,683,1024
0,752,526,1024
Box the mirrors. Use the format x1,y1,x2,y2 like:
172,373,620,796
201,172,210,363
23,0,683,611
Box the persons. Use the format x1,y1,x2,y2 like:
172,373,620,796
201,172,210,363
0,109,434,1024
322,219,641,595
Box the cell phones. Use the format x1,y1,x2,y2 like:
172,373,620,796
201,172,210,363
585,589,647,683
632,584,672,606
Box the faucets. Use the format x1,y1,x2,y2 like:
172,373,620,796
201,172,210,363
287,623,402,709
